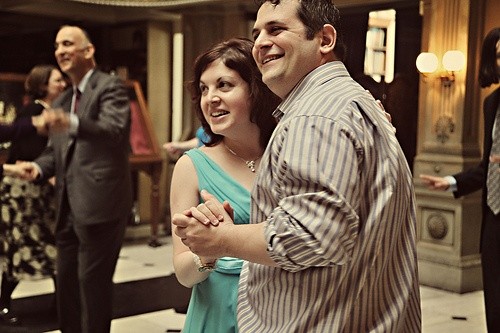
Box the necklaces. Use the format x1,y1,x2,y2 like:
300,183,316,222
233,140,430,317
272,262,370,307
221,138,268,173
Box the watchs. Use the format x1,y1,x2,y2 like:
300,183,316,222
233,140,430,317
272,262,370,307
191,253,219,274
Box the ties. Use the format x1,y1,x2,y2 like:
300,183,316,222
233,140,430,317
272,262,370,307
73,89,83,115
486,105,500,215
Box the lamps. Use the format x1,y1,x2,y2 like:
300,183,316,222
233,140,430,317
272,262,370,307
416,51,465,88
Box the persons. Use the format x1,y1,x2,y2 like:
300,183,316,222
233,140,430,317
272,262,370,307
172,0,422,333
418,27,500,333
170,37,397,333
0,64,66,325
2,26,133,333
